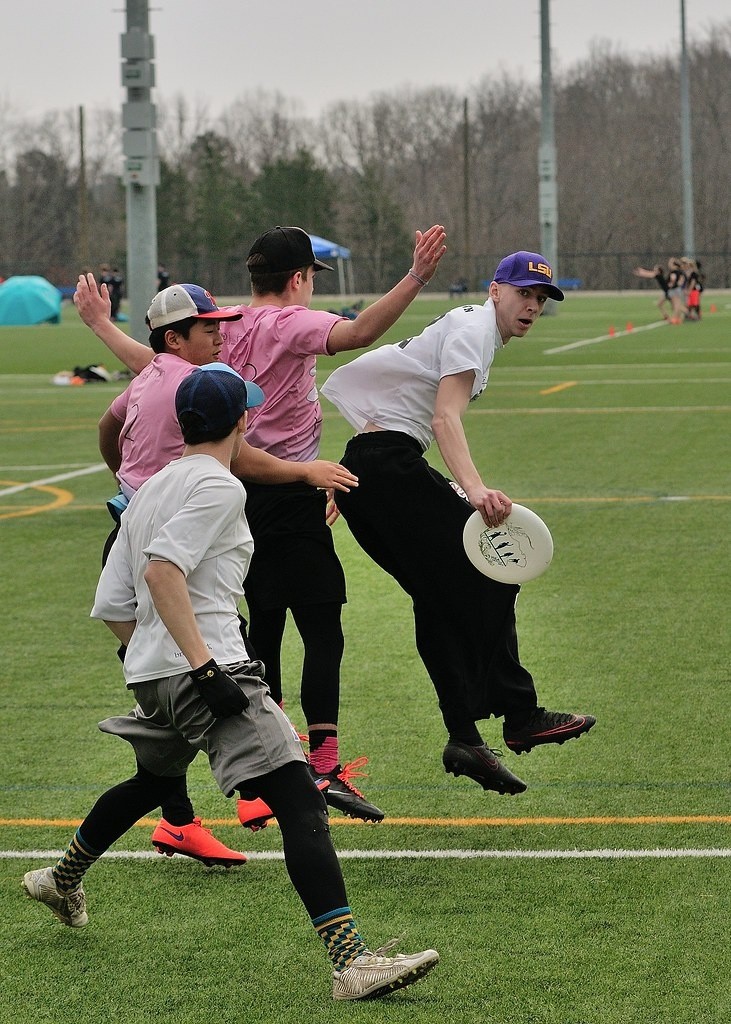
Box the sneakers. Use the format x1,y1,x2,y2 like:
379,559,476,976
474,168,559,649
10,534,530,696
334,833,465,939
152,817,247,868
304,757,384,823
333,931,439,1001
502,707,597,755
237,779,330,831
442,742,527,796
21,867,88,927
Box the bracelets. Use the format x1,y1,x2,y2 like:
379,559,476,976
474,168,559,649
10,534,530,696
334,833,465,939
408,273,426,286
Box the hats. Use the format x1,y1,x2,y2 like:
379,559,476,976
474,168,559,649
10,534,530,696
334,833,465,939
174,362,266,431
493,250,564,301
248,226,334,276
147,283,243,331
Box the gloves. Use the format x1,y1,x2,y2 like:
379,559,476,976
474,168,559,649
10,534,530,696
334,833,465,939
189,657,250,720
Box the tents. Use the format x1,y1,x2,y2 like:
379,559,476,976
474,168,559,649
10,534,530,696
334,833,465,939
309,236,355,308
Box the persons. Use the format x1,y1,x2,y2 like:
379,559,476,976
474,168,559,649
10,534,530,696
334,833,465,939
635,257,705,324
320,251,595,795
19,371,438,999
100,269,124,321
71,224,448,823
98,284,357,866
157,265,167,293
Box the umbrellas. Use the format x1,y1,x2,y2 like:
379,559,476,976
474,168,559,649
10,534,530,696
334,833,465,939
0,276,62,325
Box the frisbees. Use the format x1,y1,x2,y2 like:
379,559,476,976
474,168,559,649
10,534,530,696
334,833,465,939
461,501,554,585
